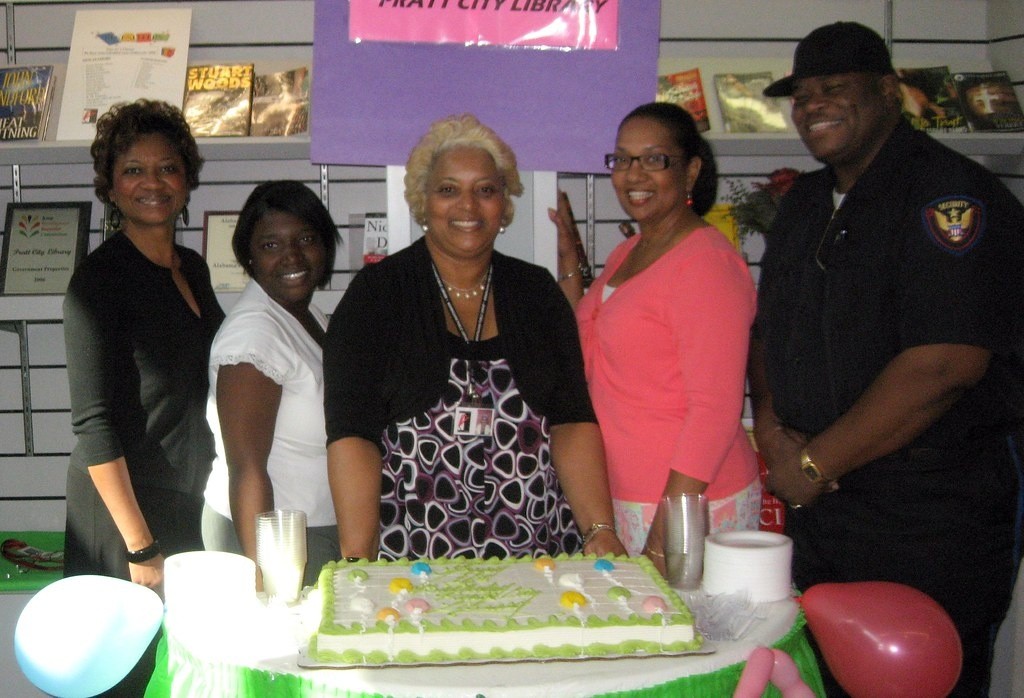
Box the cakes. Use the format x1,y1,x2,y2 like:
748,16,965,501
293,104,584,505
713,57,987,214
308,551,706,665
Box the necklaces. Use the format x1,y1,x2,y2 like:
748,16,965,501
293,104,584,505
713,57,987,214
442,267,489,298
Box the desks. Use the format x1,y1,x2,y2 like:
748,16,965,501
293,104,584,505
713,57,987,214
0,531,66,594
143,594,827,698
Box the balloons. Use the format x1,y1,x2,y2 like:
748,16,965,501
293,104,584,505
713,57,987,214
14,574,164,698
796,582,963,698
734,647,816,698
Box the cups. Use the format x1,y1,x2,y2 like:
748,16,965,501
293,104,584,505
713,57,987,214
662,491,707,590
252,510,308,602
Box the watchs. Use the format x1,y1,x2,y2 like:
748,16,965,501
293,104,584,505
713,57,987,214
800,445,834,485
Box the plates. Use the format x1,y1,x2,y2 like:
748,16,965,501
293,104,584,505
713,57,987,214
164,551,255,656
702,528,793,602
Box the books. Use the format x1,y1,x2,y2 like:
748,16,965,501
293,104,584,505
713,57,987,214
183,59,310,138
714,71,790,134
0,65,56,141
655,68,711,134
896,65,1024,134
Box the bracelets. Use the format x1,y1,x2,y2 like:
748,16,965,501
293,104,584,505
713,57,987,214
581,523,616,548
125,537,161,562
558,267,582,283
645,542,664,557
347,557,359,562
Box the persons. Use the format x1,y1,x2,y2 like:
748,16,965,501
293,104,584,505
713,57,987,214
748,20,1024,698
201,182,343,592
323,116,630,563
548,101,762,580
61,99,227,698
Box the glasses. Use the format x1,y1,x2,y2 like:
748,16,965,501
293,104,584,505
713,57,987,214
604,153,691,172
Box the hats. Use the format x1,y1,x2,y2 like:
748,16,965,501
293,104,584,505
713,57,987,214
762,21,893,97
